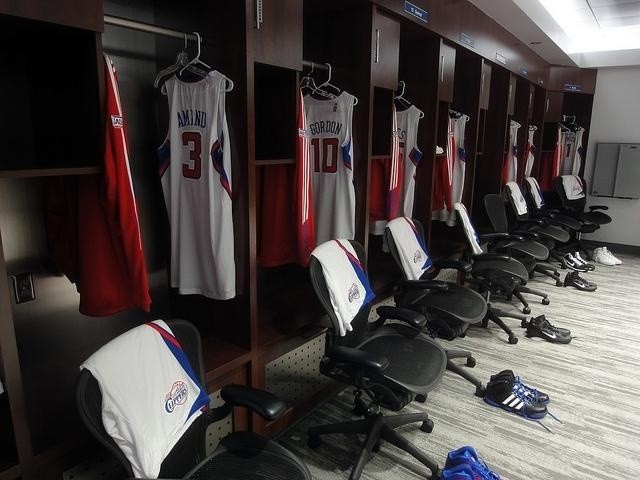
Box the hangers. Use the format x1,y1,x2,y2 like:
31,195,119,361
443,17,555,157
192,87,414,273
298,62,359,107
450,107,471,123
394,80,425,119
558,115,585,134
154,29,234,95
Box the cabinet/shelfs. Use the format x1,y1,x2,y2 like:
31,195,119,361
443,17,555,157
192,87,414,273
537,89,594,192
0,1,549,478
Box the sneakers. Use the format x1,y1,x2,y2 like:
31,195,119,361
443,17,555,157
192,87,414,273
563,251,597,291
445,446,500,480
593,248,615,266
440,463,485,480
602,246,622,265
490,369,550,405
530,314,570,334
482,377,563,434
527,319,572,344
